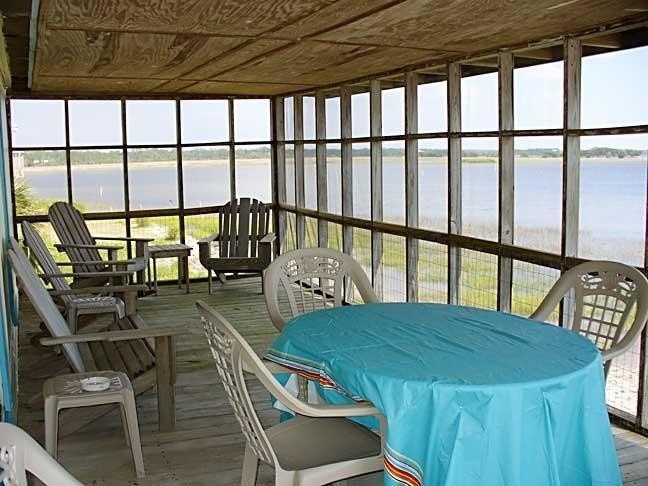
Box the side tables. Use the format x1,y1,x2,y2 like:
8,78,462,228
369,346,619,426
145,242,192,292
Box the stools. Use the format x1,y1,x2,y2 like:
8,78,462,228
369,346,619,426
43,370,145,480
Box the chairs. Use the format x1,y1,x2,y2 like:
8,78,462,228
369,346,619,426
529,259,648,379
0,422,86,486
50,201,154,298
264,247,385,332
196,197,278,295
194,299,386,486
8,237,176,434
22,219,135,315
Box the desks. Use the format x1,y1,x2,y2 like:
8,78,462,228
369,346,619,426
263,302,623,486
